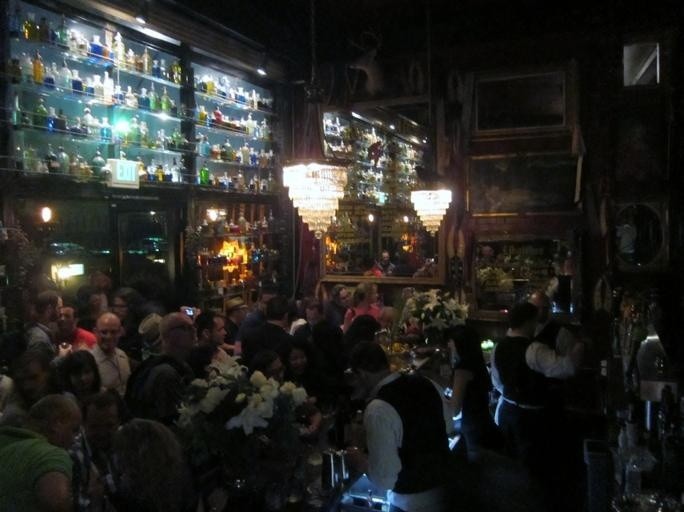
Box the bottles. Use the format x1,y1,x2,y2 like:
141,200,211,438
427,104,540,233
322,113,431,242
624,451,645,498
5,2,281,195
610,302,647,369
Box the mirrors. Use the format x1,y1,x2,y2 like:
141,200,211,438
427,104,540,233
316,94,448,287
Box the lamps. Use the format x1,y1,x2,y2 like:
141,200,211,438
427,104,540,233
280,0,355,241
408,0,453,237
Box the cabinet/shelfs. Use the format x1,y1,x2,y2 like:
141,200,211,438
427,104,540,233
322,103,428,181
0,0,278,202
139,181,285,313
474,235,572,322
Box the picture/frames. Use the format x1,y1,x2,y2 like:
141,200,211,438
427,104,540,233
471,69,574,142
464,148,585,219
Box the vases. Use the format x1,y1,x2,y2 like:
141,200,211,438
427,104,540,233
428,339,454,389
217,439,269,511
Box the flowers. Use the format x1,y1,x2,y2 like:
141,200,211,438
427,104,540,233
391,282,472,347
167,356,317,462
